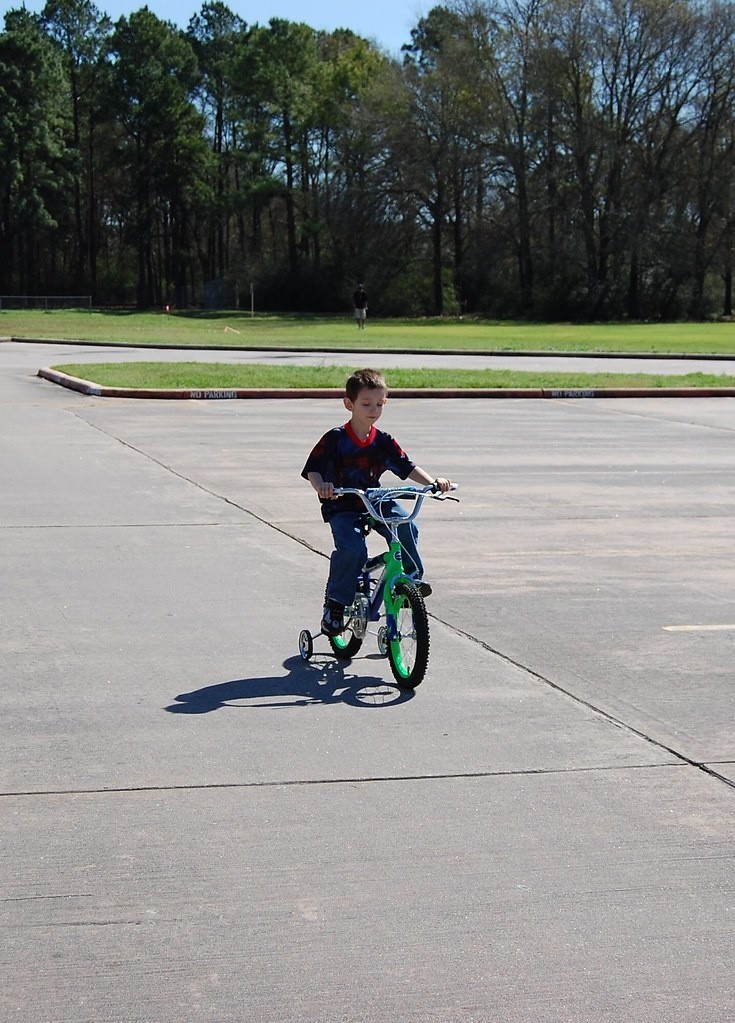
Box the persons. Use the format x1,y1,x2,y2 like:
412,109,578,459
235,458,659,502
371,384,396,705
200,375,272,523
352,284,369,331
301,366,452,637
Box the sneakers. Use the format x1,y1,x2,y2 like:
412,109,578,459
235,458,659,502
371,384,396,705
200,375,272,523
395,578,432,608
321,604,344,636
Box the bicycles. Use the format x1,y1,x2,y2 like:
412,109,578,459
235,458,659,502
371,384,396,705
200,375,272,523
297,481,460,690
